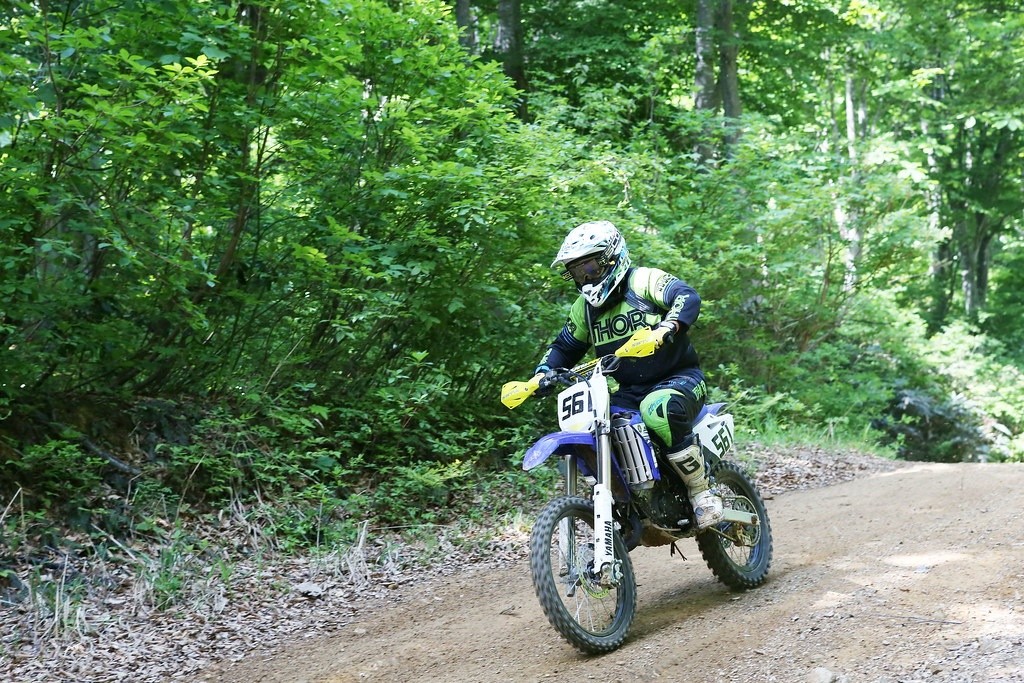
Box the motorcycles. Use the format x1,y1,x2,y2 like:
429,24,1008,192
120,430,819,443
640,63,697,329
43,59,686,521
501,326,773,655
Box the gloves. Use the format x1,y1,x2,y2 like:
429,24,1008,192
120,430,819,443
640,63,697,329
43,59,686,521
528,373,556,398
652,321,676,350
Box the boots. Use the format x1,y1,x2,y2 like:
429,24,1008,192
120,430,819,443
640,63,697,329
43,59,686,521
585,475,622,544
656,432,725,529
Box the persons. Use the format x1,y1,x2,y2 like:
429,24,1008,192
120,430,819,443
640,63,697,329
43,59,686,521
530,221,724,529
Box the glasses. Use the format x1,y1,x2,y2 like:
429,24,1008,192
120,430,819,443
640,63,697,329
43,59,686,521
568,255,605,285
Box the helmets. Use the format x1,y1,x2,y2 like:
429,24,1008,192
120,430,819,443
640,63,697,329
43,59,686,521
550,220,631,308
